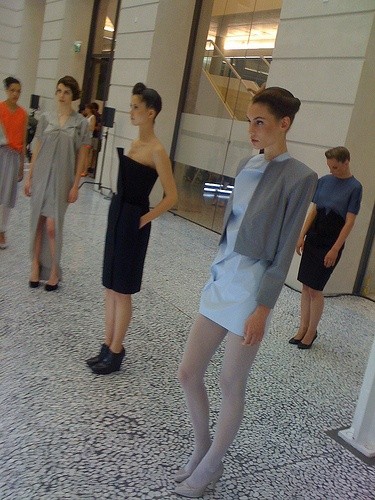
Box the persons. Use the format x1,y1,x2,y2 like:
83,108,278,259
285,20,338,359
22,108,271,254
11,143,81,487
0,76,28,248
288,146,363,349
85,83,180,375
173,87,318,498
78,103,101,176
24,76,92,291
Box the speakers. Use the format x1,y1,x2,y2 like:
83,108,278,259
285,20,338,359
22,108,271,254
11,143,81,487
30,95,40,109
102,107,116,127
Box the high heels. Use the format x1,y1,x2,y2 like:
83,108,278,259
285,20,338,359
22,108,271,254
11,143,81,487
88,344,109,364
91,347,125,373
298,330,317,349
288,337,300,344
175,439,213,482
175,461,224,498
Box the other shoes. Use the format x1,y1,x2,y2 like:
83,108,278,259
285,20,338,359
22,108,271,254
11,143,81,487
45,284,58,291
30,281,40,288
80,172,88,176
0,243,8,249
